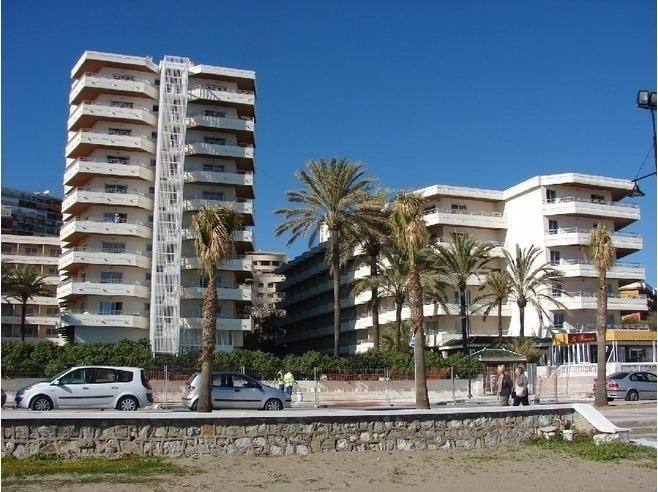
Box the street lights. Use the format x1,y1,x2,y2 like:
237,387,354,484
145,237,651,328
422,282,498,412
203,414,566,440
628,91,657,198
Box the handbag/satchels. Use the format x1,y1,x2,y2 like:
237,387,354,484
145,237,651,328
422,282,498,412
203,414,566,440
516,384,527,397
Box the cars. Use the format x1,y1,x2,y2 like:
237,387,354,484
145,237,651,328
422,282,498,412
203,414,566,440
181,370,292,410
15,365,153,411
593,371,657,401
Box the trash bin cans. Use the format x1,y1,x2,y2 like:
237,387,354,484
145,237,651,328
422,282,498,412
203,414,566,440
530,398,540,405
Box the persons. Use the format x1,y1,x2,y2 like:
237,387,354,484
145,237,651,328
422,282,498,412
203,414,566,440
513,367,529,406
496,364,513,406
276,369,284,391
283,369,295,396
